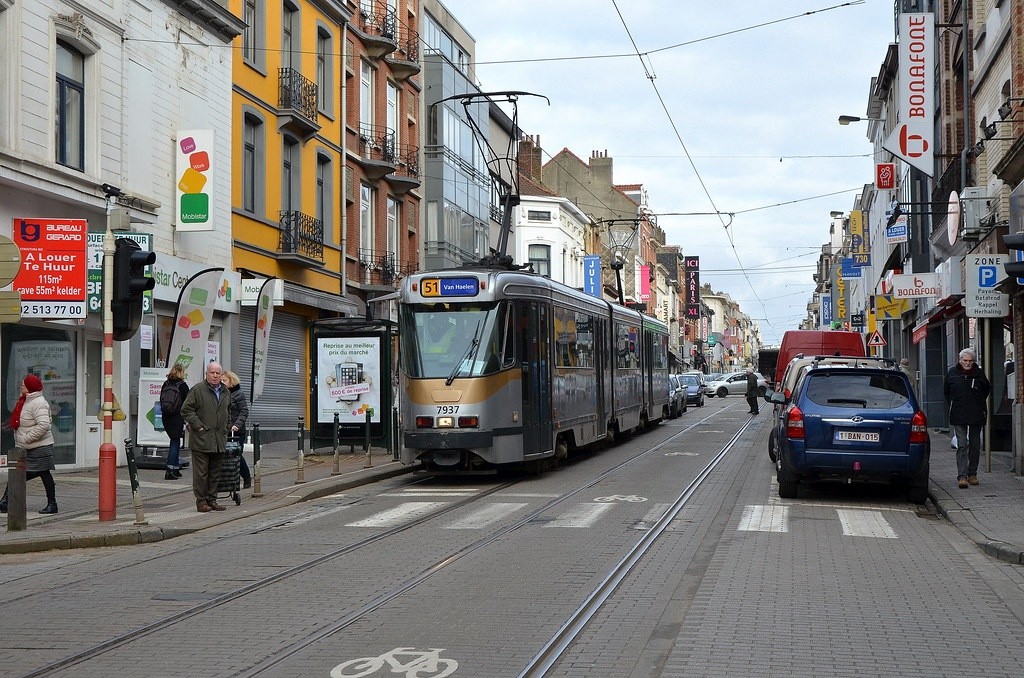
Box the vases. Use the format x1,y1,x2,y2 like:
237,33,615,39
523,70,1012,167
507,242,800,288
363,25,378,34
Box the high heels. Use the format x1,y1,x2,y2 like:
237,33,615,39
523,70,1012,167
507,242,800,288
173,470,182,477
165,471,178,480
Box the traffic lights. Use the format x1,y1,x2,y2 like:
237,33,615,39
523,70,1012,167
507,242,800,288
116,243,156,301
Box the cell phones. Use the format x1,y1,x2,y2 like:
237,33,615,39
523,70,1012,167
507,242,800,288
335,362,363,402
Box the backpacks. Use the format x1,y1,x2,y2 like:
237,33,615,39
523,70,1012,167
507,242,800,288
161,380,185,414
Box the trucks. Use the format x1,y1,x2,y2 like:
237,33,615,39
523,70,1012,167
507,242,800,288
773,329,866,392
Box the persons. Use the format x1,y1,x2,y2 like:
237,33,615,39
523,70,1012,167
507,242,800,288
160,364,190,480
220,371,252,489
883,358,900,371
0,375,58,514
944,348,991,489
418,304,456,356
744,369,759,415
180,362,232,512
899,358,914,389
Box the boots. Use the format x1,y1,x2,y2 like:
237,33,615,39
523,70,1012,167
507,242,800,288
39,478,58,513
0,484,12,512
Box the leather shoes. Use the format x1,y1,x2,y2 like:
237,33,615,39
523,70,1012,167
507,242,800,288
197,504,212,512
209,501,226,510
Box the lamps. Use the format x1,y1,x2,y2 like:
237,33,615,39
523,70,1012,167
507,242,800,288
885,199,962,229
984,120,1024,141
838,115,887,127
998,98,1024,121
973,138,1016,158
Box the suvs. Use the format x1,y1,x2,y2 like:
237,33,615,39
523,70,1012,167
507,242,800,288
767,352,932,505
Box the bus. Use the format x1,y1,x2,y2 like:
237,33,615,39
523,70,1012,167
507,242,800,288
393,88,669,480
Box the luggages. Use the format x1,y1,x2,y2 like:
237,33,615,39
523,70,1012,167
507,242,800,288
216,428,242,505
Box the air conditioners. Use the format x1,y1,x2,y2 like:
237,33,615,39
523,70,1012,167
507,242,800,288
958,186,986,238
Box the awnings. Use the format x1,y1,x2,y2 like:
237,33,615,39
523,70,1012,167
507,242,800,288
669,350,691,366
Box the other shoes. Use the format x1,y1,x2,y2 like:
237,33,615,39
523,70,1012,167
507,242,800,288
748,410,759,414
968,475,979,485
244,477,251,488
958,477,968,488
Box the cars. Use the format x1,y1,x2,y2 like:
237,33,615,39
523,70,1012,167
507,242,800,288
703,371,771,399
663,370,722,418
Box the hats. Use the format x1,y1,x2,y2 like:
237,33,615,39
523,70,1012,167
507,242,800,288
24,375,43,393
